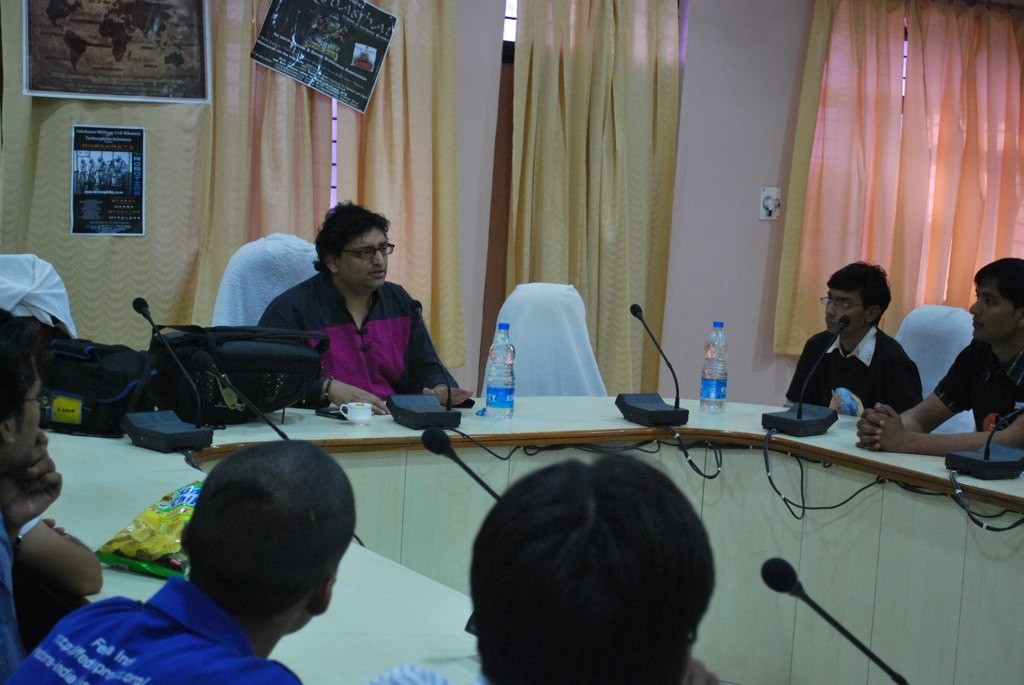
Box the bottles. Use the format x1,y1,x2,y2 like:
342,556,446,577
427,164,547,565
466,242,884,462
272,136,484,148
485,323,515,420
699,321,728,413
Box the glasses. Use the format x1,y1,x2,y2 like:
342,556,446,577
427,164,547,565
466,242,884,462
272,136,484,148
820,295,870,311
20,391,55,409
329,243,394,260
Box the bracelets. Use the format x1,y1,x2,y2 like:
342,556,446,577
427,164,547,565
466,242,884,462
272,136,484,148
323,376,335,402
13,534,24,558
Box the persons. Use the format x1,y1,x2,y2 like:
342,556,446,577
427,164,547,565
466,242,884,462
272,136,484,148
0,336,104,685
257,201,474,415
2,440,358,684
855,256,1024,457
366,449,721,685
785,260,925,419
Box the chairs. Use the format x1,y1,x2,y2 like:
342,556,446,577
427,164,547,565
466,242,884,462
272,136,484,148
894,304,976,433
480,283,608,398
211,233,318,326
0,254,77,339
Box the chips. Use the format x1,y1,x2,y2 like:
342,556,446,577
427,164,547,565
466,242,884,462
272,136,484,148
100,516,188,562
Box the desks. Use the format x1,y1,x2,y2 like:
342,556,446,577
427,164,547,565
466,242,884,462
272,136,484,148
40,396,1024,685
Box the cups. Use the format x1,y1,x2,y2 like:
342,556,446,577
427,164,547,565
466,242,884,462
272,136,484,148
340,402,372,425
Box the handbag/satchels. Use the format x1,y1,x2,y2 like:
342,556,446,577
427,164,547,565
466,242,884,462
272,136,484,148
38,338,143,438
148,324,330,425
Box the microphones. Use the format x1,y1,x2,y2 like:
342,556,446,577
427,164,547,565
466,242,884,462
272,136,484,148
387,300,462,428
761,557,909,685
614,304,689,426
761,314,852,437
422,428,501,500
119,297,213,452
191,349,289,441
